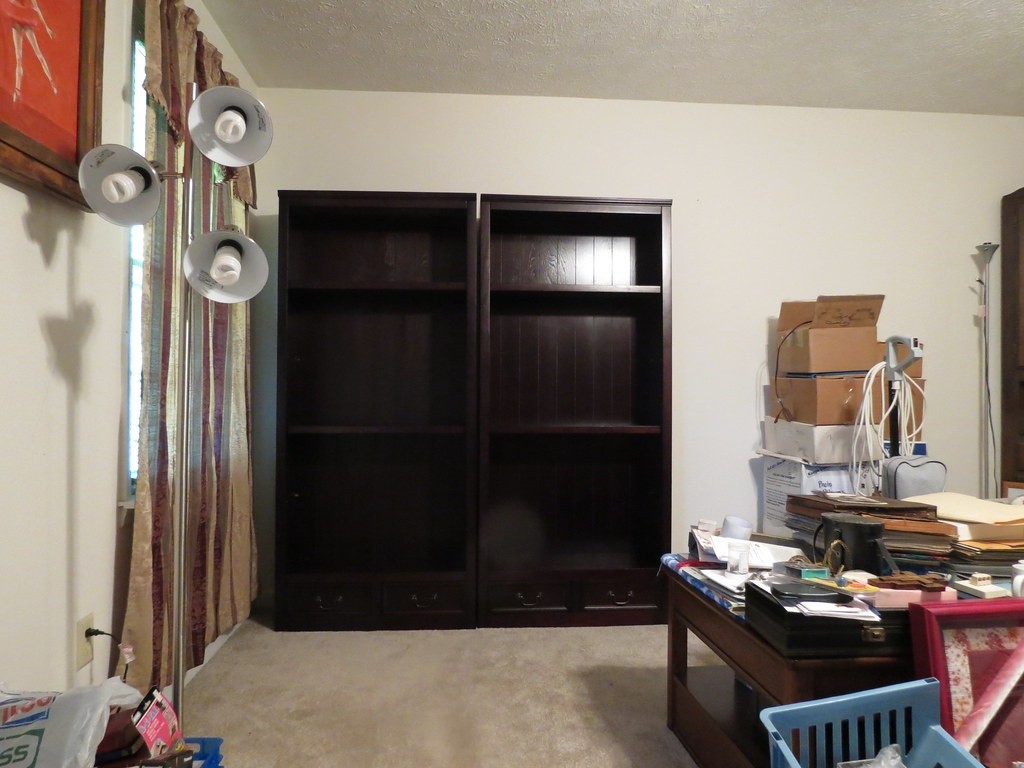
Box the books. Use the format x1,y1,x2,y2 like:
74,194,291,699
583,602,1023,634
784,492,1024,579
691,529,823,569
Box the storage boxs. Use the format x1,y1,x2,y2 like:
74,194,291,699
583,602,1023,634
745,582,913,657
759,677,986,768
761,294,927,539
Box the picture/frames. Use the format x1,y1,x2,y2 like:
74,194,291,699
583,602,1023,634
1002,481,1024,499
908,598,1024,768
0,0,107,213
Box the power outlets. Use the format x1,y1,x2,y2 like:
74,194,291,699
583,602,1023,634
76,613,94,671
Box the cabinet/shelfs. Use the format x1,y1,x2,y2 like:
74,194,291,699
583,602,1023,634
480,193,672,628
273,191,480,629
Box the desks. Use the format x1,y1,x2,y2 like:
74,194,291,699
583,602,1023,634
658,552,1024,768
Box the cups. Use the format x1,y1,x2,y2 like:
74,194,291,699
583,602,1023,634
719,516,753,540
1011,563,1024,598
727,541,751,575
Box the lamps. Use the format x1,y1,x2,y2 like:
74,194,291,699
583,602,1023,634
78,144,184,225
188,87,273,167
184,225,268,303
